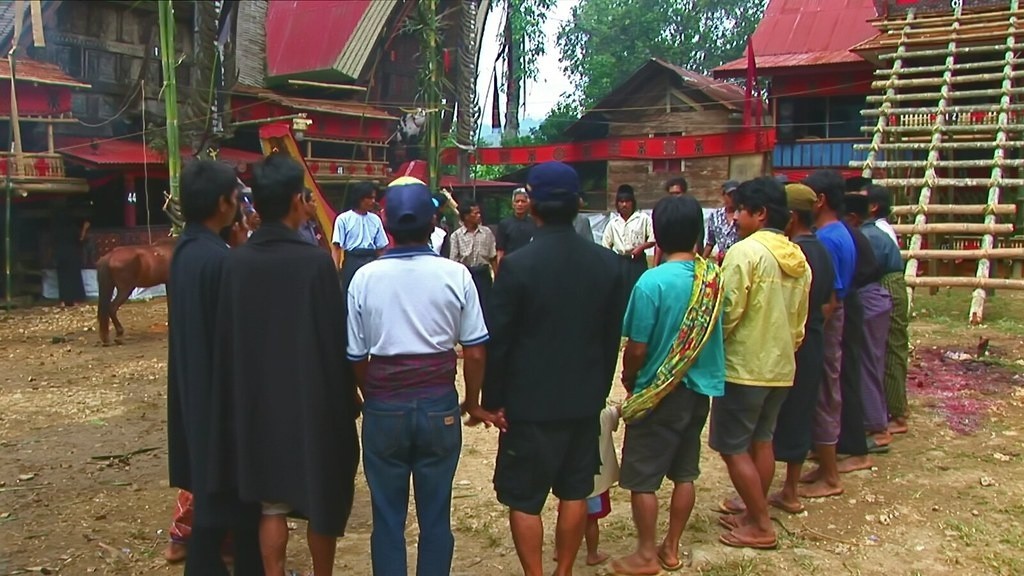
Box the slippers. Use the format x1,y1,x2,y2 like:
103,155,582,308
595,558,666,576
719,527,777,549
656,547,685,570
718,494,750,514
718,513,742,531
767,488,806,514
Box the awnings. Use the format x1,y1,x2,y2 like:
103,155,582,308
35,136,267,178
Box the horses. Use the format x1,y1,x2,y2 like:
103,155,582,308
91,232,182,348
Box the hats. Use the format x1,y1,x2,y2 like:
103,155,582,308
386,176,433,236
525,161,580,201
785,182,818,212
722,180,741,194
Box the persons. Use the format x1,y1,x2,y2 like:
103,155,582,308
47,193,93,308
167,151,911,576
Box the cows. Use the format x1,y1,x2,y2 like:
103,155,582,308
391,105,441,146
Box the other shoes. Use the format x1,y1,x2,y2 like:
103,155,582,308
862,437,890,454
